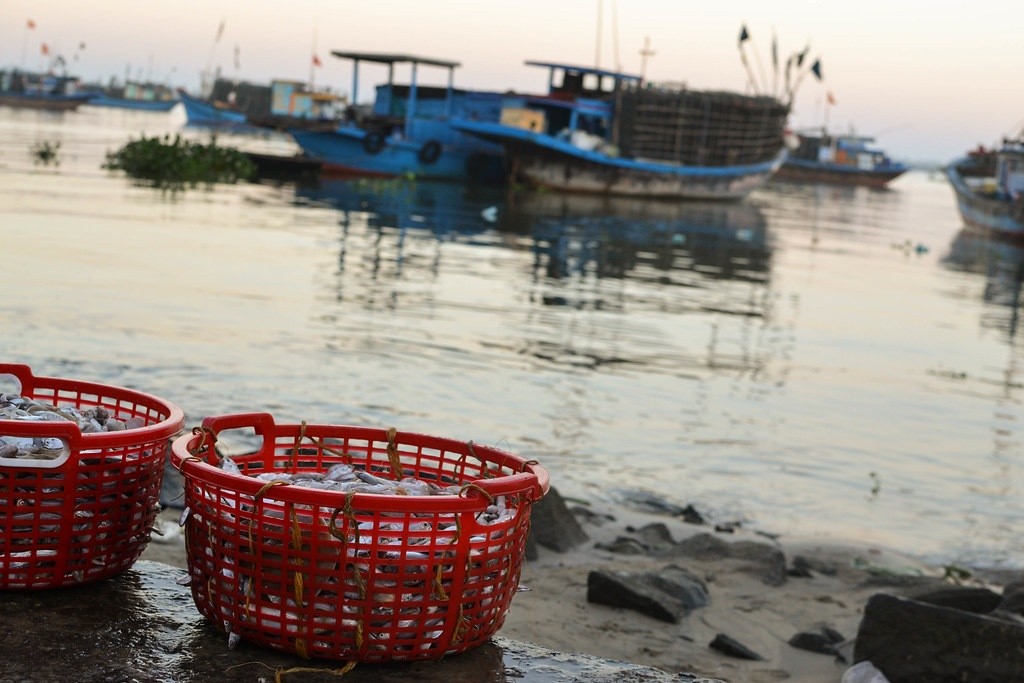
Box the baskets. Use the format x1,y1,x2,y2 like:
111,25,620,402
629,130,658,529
170,412,550,664
0,362,185,585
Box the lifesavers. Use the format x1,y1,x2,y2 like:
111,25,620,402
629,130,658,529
363,129,386,154
419,140,441,165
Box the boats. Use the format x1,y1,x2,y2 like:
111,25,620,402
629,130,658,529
485,169,774,291
446,17,799,200
946,124,1024,237
290,163,499,290
284,49,519,186
772,120,911,191
0,22,352,143
